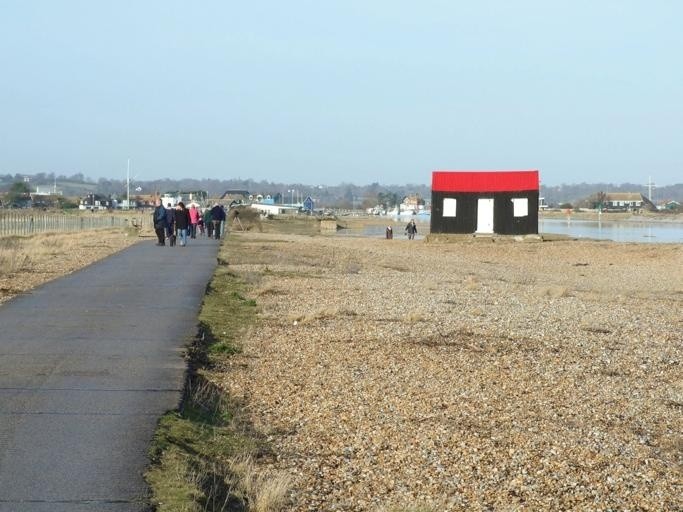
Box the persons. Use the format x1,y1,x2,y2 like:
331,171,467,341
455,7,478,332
405,219,417,240
149,197,227,246
